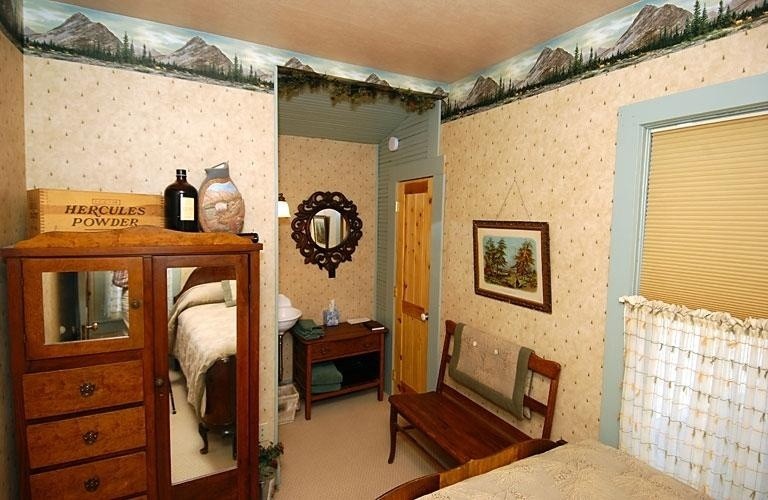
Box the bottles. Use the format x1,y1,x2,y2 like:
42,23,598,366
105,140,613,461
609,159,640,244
163,168,200,233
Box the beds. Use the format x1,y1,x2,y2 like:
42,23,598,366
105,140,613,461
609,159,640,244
173,268,241,462
373,440,718,500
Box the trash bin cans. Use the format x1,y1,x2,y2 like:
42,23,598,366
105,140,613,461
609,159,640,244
278,383,299,426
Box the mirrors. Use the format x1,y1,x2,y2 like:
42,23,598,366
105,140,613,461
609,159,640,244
38,269,132,345
291,191,363,279
167,265,241,487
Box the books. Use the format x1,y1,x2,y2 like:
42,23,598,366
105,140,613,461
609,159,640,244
363,320,385,331
345,316,370,324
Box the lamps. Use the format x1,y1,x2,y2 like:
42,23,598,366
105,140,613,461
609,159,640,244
278,193,292,219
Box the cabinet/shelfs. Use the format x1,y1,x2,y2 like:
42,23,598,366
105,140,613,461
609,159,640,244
292,334,386,421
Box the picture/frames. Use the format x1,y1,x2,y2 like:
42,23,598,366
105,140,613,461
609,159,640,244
472,221,551,315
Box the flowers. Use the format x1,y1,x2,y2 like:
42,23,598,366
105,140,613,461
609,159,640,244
257,441,285,482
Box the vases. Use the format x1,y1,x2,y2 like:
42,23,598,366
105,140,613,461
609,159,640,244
261,476,276,500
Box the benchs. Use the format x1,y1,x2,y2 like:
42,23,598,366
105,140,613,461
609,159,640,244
386,320,561,470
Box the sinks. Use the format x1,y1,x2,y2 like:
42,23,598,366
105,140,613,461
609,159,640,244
277,306,301,333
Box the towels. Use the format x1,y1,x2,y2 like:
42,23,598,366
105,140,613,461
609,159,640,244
292,318,324,342
